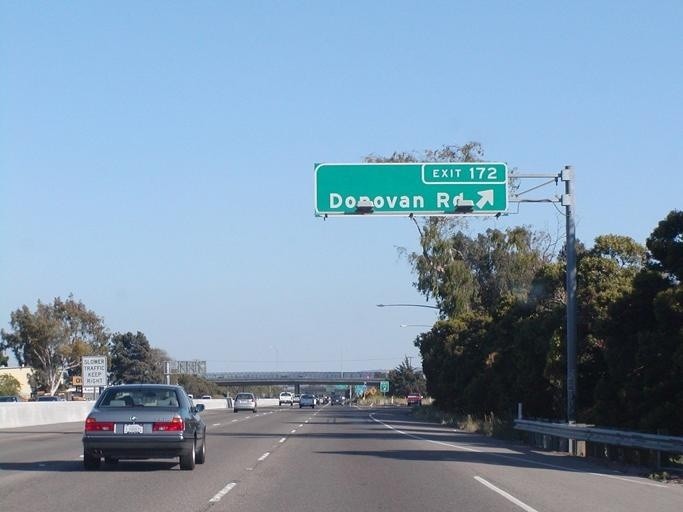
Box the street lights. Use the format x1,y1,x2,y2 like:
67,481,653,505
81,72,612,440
398,323,434,328
374,303,440,310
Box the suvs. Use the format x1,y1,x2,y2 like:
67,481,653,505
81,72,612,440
231,391,259,414
405,392,425,407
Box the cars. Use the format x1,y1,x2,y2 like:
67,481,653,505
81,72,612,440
0,391,90,404
200,394,213,399
275,389,346,409
186,393,195,400
80,383,208,472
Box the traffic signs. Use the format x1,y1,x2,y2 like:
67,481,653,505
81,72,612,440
380,381,390,392
312,161,511,216
81,354,108,385
354,384,366,393
